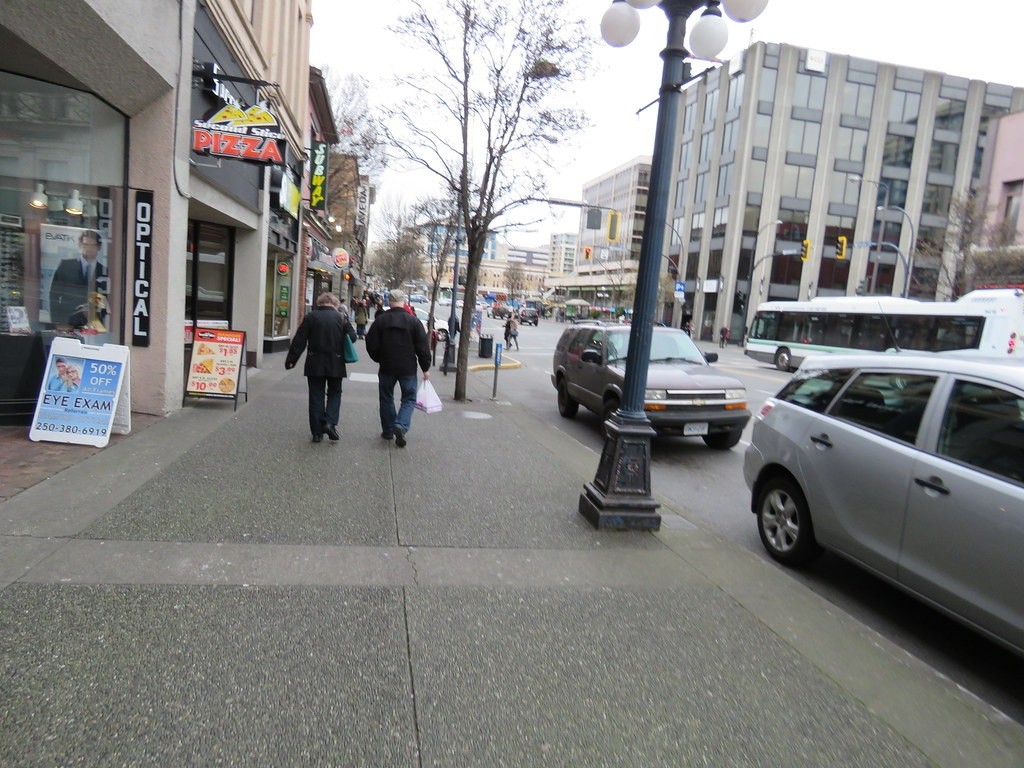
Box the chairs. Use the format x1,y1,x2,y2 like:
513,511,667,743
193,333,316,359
951,392,1024,486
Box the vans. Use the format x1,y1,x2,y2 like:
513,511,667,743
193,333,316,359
517,307,539,326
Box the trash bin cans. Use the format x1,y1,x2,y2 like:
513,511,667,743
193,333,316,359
478,334,493,357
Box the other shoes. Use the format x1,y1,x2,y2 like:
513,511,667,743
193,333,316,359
323,423,341,440
380,429,393,439
392,425,407,449
312,434,323,441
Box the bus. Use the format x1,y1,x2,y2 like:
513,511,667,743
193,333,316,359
742,288,1024,374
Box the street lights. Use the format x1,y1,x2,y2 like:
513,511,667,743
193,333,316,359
575,0,775,530
743,220,784,341
848,175,889,296
877,203,916,298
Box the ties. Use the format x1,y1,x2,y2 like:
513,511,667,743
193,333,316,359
85,265,91,283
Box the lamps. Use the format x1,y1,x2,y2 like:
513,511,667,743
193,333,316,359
64,189,83,215
82,199,97,218
29,183,48,208
48,196,63,211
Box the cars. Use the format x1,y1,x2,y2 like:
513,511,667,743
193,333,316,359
410,295,429,304
438,299,512,319
742,347,1024,667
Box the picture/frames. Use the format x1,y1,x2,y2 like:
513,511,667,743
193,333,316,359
4,306,32,334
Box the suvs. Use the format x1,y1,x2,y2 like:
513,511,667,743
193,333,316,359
551,319,752,454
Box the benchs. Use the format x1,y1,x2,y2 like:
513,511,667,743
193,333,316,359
837,385,931,443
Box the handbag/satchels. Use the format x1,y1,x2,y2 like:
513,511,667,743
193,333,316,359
511,329,519,336
340,310,359,363
413,377,442,414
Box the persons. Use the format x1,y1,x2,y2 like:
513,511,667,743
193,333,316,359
448,315,459,335
427,313,435,336
48,357,81,392
560,309,564,321
14,309,25,324
366,290,431,447
502,314,519,350
285,292,357,441
49,231,107,324
577,312,581,319
719,326,730,348
537,307,548,319
685,323,690,336
339,299,357,343
351,287,389,340
619,314,624,324
405,300,416,316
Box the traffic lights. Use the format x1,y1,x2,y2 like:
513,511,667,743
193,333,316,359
801,239,810,261
584,246,593,261
835,236,847,260
